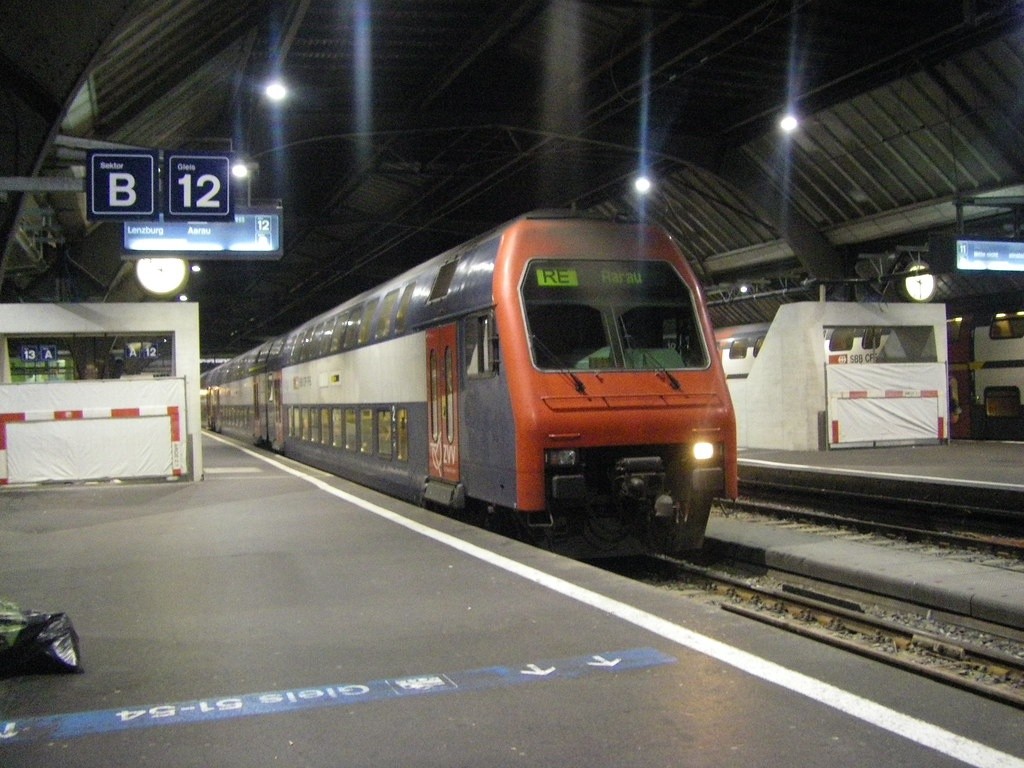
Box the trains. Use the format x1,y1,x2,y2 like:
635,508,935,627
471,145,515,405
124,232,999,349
197,206,743,579
705,306,1024,440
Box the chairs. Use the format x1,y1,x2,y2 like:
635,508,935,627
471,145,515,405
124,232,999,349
583,344,682,370
